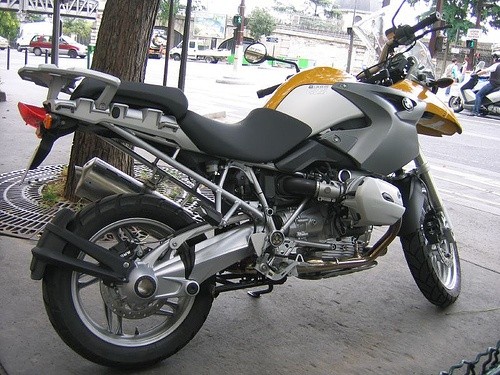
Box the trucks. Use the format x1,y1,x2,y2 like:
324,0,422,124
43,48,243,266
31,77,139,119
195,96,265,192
15,21,54,53
169,40,232,64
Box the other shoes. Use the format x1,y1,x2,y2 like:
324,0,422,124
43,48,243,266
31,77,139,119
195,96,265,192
467,112,479,116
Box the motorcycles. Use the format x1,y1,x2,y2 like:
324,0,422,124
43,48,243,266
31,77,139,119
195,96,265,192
18,1,464,365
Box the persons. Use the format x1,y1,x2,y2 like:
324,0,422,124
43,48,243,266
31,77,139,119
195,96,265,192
443,58,459,94
159,44,163,58
467,51,500,116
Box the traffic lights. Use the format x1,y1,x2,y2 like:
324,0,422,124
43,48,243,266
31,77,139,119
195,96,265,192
231,15,242,27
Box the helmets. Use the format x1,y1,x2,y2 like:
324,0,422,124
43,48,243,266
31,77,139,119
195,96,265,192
491,51,500,57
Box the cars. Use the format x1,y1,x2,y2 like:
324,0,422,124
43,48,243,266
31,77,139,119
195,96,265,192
28,34,89,58
0,36,9,51
147,29,166,59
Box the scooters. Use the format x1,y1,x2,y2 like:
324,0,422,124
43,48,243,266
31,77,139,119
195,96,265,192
445,60,500,115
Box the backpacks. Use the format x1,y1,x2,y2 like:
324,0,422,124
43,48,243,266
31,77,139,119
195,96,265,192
446,64,454,77
